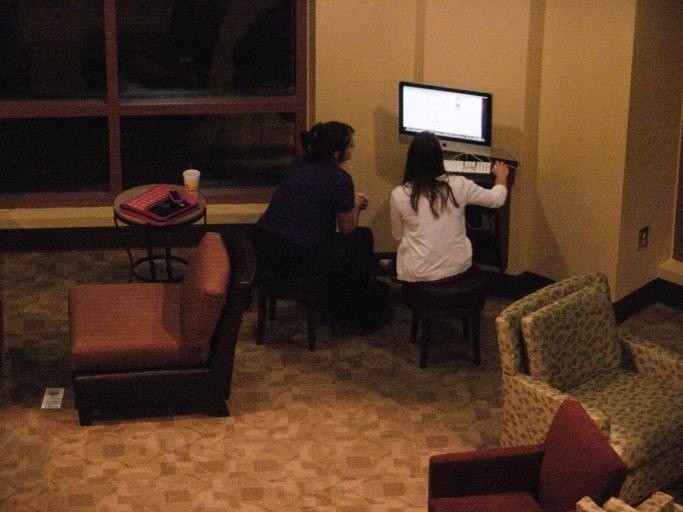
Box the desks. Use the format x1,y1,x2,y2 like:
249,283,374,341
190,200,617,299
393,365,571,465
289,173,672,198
404,151,517,298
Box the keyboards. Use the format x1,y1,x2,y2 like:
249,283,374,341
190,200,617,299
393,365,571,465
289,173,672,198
443,159,491,176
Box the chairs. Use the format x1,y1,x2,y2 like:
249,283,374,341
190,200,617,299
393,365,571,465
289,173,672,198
576,490,682,512
496,273,683,506
427,401,629,512
68,228,257,426
255,230,345,351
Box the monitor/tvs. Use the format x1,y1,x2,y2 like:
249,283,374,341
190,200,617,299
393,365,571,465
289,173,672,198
397,80,494,147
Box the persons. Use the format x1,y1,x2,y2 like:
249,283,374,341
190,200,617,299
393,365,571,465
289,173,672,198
251,118,387,324
386,130,508,316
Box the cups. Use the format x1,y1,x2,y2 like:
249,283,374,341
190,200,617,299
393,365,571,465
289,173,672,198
183,169,201,196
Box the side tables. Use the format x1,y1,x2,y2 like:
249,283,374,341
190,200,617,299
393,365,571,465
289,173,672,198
112,183,206,282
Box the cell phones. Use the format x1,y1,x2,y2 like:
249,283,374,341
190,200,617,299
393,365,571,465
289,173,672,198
168,190,182,203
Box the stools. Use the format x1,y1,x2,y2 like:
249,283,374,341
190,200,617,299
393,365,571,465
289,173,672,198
400,264,489,369
